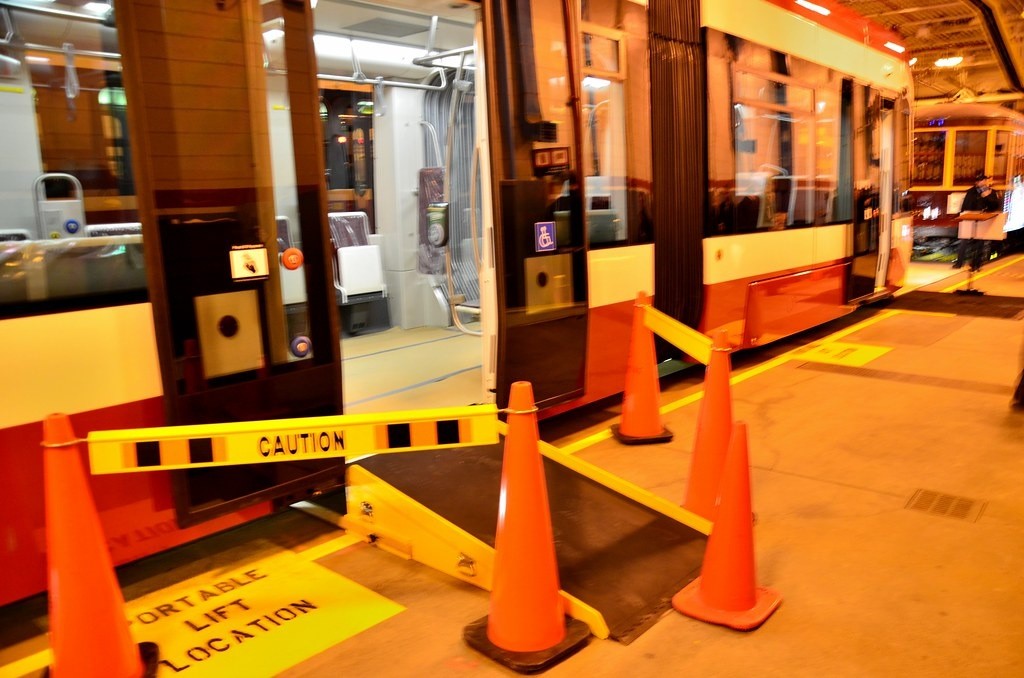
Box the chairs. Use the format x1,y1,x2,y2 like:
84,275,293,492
84,221,146,290
328,211,391,304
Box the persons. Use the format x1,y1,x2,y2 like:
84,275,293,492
951,175,1000,271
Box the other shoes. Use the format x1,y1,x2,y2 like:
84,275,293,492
953,261,963,268
971,262,980,271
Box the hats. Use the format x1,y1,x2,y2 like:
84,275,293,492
974,169,985,180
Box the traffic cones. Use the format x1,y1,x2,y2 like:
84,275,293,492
607,290,675,447
671,420,783,631
678,327,755,526
461,378,592,674
35,414,162,678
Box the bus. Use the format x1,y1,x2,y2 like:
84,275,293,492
911,100,1024,257
0,0,916,611
